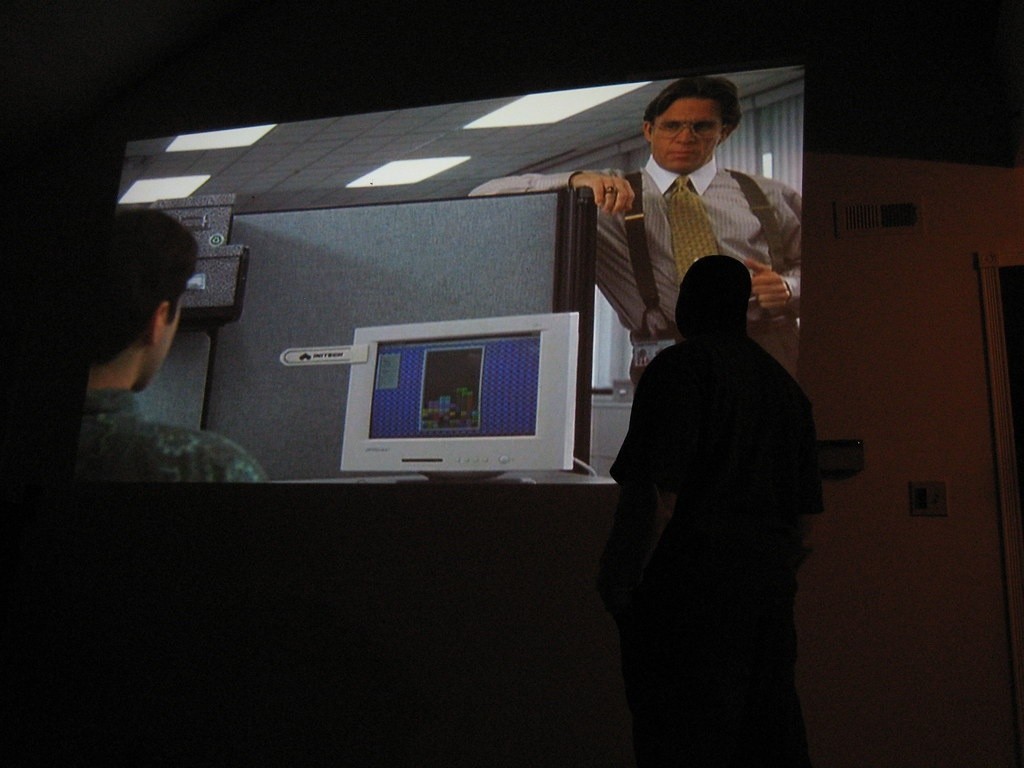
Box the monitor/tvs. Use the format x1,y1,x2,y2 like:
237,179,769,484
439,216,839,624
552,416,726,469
340,313,580,474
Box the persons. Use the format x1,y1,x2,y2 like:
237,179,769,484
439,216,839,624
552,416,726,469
71,209,268,484
598,255,824,768
467,76,802,386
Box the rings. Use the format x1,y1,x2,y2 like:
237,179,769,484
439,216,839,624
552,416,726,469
605,186,614,193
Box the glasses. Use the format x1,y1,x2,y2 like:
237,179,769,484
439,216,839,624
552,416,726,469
650,122,727,139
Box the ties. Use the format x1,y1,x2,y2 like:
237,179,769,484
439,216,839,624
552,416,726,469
668,176,718,281
